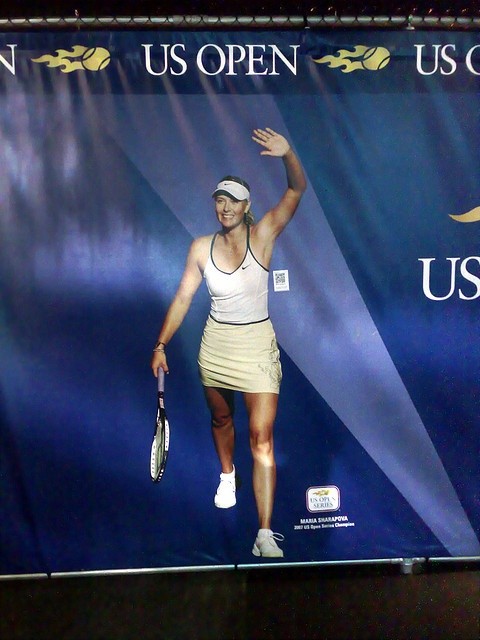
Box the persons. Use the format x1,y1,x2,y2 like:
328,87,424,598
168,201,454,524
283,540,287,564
149,126,306,559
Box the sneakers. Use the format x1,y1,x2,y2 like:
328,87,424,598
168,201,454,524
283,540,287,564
213,463,237,509
252,532,285,559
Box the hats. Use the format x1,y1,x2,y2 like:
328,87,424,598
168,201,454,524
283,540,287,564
212,180,250,203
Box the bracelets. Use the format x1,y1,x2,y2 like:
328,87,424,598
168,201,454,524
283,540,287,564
151,349,165,352
152,339,166,349
